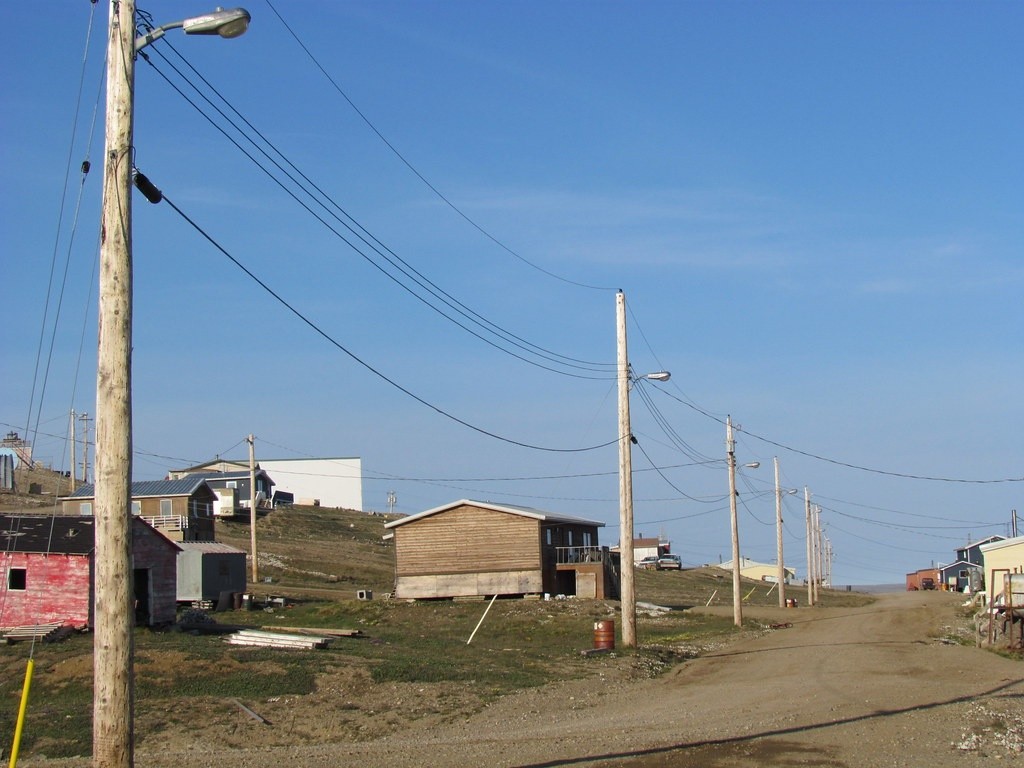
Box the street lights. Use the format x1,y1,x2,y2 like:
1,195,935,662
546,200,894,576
617,368,675,647
90,4,254,768
728,462,761,628
776,488,799,607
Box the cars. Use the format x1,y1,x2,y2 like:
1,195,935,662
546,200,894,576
640,556,659,566
921,577,935,591
658,553,682,570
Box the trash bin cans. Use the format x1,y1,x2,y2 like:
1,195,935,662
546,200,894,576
937,583,947,590
593,618,615,650
785,599,798,607
847,585,851,591
233,592,255,610
